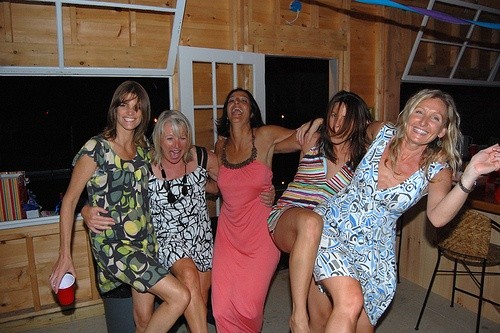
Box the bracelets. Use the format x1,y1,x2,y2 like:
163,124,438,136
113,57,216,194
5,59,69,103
459,174,477,194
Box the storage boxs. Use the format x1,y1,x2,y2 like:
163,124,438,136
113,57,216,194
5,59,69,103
0,171,26,222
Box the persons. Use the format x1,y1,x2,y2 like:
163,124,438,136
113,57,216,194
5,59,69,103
48,80,191,332
296,88,500,333
80,109,275,333
205,87,296,333
218,90,373,333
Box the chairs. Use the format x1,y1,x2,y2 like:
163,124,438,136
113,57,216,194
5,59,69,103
414,210,500,333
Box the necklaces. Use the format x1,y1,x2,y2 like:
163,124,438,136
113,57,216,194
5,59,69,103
158,160,188,206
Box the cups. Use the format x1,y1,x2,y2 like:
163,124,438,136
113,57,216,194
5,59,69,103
53,273,75,307
